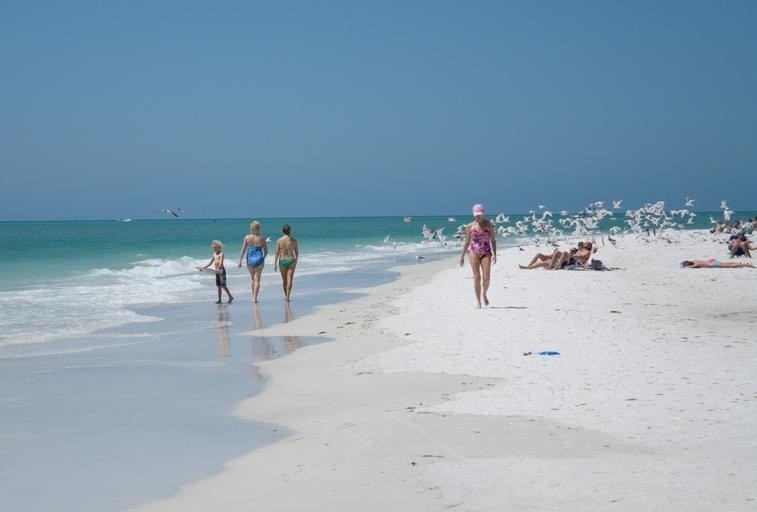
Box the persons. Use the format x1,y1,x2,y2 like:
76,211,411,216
517,241,593,270
458,204,497,309
197,239,234,304
273,224,298,302
246,304,272,383
280,300,302,350
680,259,755,269
709,216,756,261
213,303,233,362
237,220,268,304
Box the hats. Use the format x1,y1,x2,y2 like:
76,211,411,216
472,203,484,217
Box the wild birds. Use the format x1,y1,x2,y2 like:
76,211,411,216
415,256,426,261
383,194,736,251
265,236,272,243
158,209,181,217
